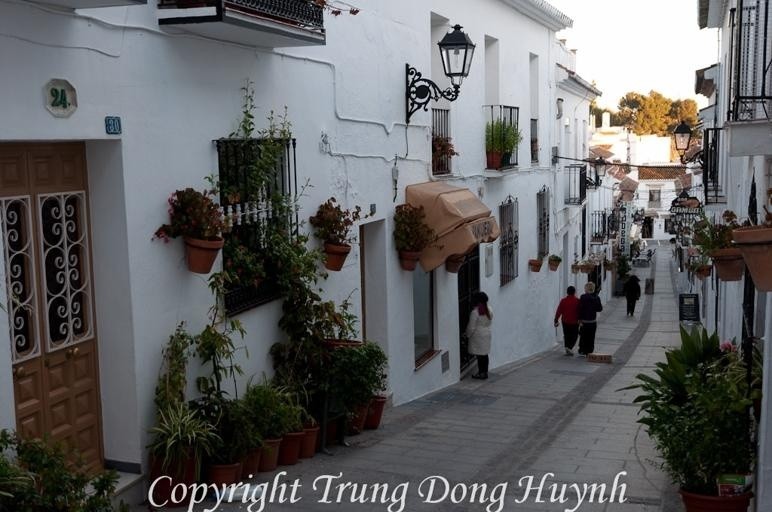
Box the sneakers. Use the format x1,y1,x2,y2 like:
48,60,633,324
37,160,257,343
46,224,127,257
563,346,574,357
471,372,489,381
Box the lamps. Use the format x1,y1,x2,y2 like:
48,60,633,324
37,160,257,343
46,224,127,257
674,120,713,165
586,156,607,189
403,23,476,124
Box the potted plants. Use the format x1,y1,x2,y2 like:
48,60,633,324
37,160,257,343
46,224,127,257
621,322,765,510
484,116,522,170
549,254,562,271
148,280,390,507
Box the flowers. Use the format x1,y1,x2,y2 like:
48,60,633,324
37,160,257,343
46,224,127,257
431,134,460,160
152,185,228,243
681,209,749,254
390,203,444,252
309,197,366,244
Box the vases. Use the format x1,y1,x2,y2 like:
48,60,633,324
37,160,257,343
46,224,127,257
182,233,226,274
397,248,421,272
706,247,745,281
697,264,713,276
322,239,353,271
731,223,771,292
528,259,544,272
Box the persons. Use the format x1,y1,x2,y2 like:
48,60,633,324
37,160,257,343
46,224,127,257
623,273,643,316
574,281,602,356
552,283,580,357
462,291,494,380
